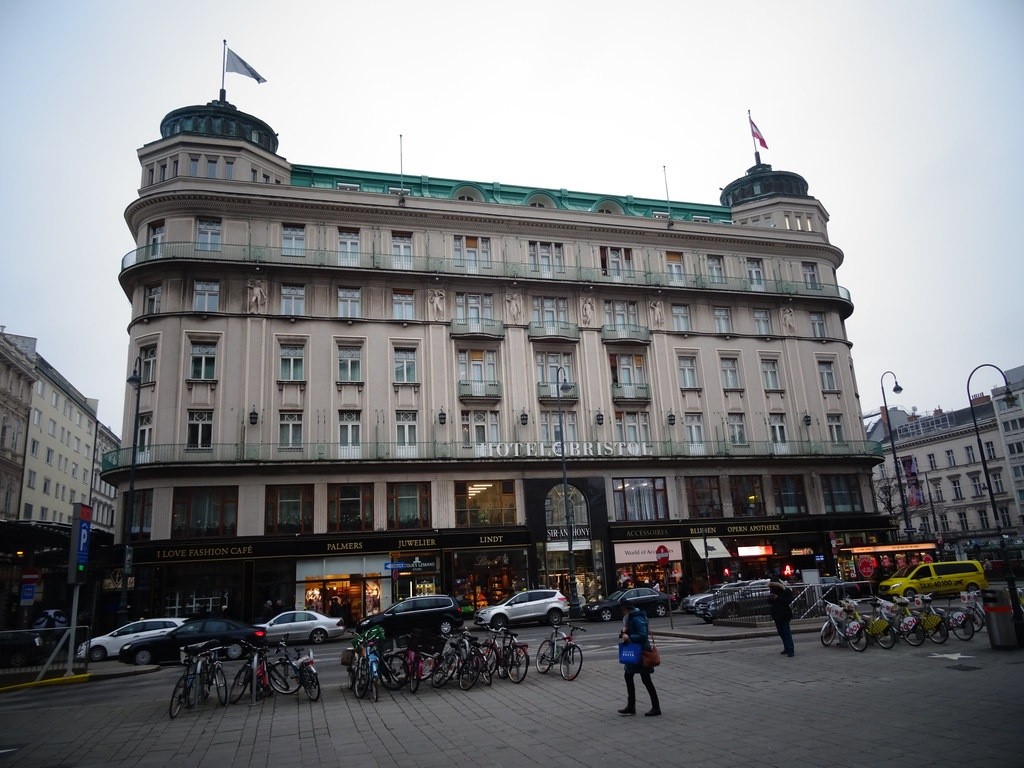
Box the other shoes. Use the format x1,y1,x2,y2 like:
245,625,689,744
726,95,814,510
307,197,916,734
645,708,662,716
618,706,636,714
780,649,787,654
788,651,794,657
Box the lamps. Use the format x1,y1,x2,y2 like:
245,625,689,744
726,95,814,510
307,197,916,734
249,406,257,425
597,410,604,426
802,410,813,426
668,412,675,425
438,408,447,425
520,406,528,425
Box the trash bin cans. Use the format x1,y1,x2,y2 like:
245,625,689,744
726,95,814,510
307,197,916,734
981,589,1016,650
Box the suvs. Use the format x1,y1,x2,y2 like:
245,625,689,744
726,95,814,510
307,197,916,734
355,593,463,637
473,589,571,630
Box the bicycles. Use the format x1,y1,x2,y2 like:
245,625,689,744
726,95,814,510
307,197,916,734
535,621,587,680
341,623,530,702
819,587,1024,652
229,633,321,704
170,640,227,719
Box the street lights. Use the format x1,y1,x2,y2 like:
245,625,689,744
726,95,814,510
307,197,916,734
556,366,583,619
117,356,142,622
967,364,1024,650
881,371,915,543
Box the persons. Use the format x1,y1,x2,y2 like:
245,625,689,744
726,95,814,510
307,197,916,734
222,605,230,619
618,601,661,716
77,615,88,642
983,558,992,582
329,598,341,618
768,579,794,657
263,600,282,623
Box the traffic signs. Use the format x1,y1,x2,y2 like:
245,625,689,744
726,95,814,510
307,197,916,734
384,562,406,570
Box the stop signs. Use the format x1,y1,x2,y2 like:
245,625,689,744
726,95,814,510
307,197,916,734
858,557,874,577
656,545,669,566
22,566,42,586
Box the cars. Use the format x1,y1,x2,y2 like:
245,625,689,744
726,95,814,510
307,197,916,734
0,628,44,669
252,610,346,645
582,587,679,622
119,618,267,665
76,618,190,662
680,577,860,624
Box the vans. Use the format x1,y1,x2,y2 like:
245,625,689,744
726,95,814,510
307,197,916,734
878,559,988,601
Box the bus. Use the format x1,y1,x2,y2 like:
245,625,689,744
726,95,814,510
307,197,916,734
838,543,937,583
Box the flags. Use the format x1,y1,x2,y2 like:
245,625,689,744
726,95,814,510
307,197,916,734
751,120,768,149
226,47,267,84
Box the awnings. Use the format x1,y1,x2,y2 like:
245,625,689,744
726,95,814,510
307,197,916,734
690,538,731,559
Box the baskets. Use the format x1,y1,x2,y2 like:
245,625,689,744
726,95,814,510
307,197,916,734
914,597,924,607
876,600,894,614
826,603,843,618
961,591,977,603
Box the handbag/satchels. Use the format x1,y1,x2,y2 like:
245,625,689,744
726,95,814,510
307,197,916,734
618,639,641,664
642,645,660,667
